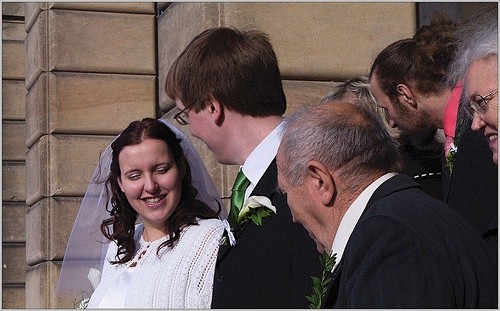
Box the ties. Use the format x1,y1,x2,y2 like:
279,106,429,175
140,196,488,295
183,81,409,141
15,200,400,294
227,171,250,230
443,133,454,164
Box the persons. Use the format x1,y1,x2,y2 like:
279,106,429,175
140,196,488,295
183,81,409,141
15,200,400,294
329,25,499,256
72,118,226,310
163,25,329,309
277,100,497,308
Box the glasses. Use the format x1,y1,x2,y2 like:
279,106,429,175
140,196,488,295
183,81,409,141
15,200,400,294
463,88,498,120
174,101,196,126
